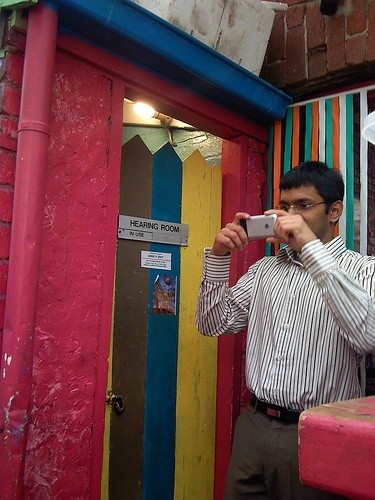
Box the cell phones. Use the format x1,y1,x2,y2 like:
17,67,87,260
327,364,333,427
240,214,277,240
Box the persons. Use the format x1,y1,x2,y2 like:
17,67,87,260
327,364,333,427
195,161,375,500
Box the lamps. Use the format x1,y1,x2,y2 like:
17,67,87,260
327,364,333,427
133,101,172,126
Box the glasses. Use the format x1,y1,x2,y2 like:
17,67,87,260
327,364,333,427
276,202,324,210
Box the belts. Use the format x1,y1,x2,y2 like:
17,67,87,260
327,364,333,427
250,396,299,425
